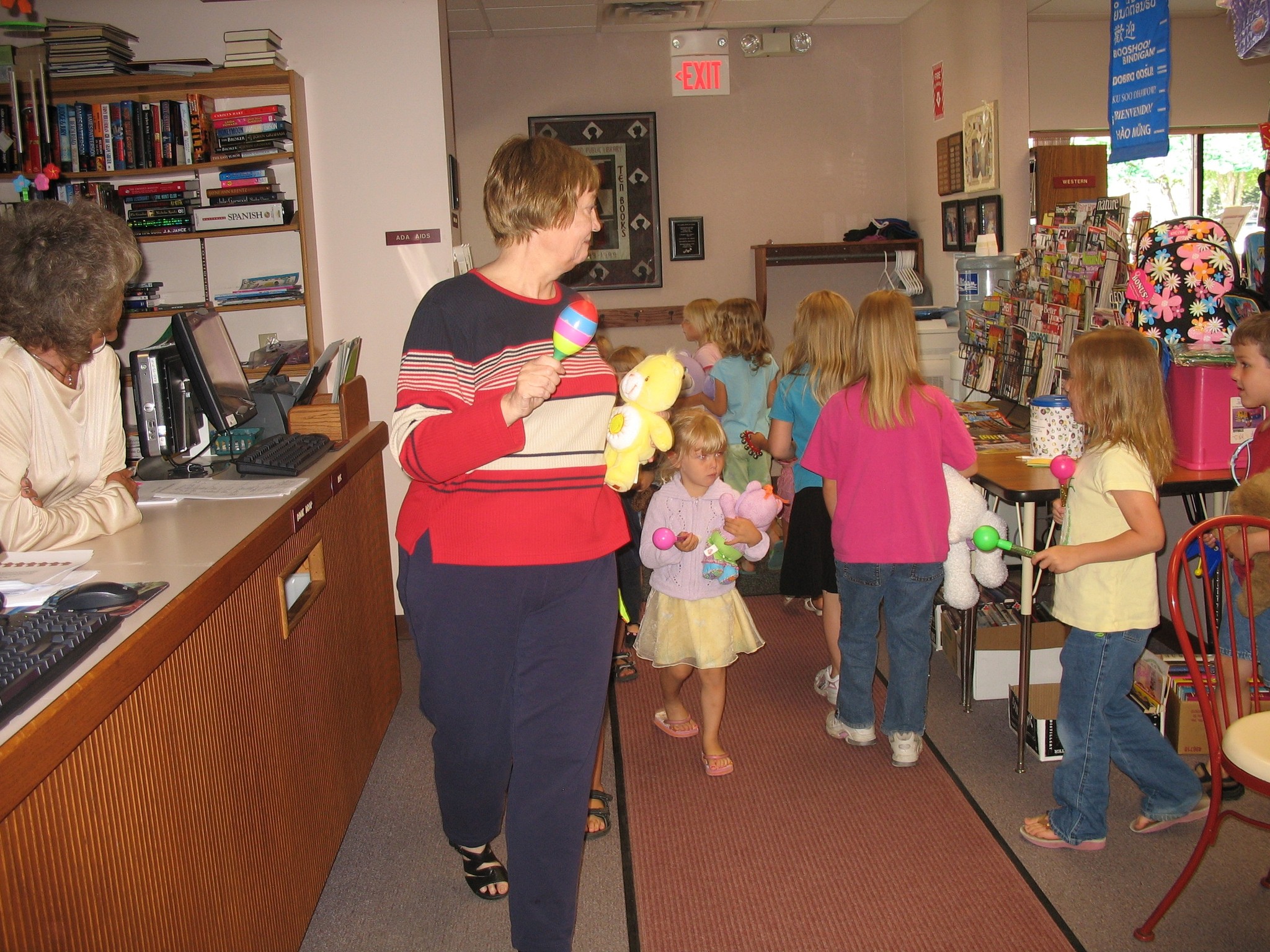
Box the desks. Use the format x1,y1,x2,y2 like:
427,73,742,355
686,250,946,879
952,400,1246,774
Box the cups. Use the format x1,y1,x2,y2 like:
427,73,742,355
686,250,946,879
975,233,999,257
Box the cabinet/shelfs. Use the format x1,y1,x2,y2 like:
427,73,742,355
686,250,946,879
1028,146,1107,228
0,70,328,394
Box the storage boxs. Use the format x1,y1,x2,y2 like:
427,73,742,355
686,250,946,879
1165,361,1267,471
931,584,1269,763
191,198,294,232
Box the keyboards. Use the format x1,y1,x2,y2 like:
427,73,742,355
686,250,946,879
0,607,121,725
234,432,336,477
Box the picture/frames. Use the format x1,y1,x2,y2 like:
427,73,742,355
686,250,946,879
526,112,663,292
977,194,1003,254
941,200,960,252
962,100,1001,193
960,198,980,251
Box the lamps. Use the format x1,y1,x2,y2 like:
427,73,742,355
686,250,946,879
668,27,730,56
739,29,812,58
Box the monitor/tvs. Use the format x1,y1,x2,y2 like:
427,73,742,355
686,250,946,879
173,307,258,434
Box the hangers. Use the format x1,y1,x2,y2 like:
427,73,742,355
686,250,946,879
876,248,924,296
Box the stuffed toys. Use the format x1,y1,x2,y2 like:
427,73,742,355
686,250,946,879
1213,469,1270,618
603,354,683,492
670,350,721,425
941,462,1010,609
702,481,783,584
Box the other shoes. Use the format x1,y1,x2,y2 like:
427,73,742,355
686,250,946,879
738,562,756,576
625,621,641,647
1260,872,1270,888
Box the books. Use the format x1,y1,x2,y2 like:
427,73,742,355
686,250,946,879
122,282,163,313
215,273,302,306
157,301,213,311
951,192,1131,453
935,577,1270,714
0,19,294,237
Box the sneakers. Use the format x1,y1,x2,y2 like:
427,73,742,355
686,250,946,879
889,731,923,768
813,664,840,705
826,711,876,746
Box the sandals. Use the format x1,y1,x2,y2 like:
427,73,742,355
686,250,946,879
584,789,613,840
1192,762,1246,801
611,652,639,683
456,842,509,900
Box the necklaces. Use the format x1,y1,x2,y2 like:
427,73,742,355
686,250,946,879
28,351,73,386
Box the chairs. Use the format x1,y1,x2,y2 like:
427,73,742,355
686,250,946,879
1131,514,1270,939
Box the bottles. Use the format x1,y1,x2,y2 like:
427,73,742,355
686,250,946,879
957,255,1016,343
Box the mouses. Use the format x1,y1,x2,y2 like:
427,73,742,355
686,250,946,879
59,581,137,609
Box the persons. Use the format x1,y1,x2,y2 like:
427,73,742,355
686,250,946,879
1192,312,1270,801
0,197,144,552
1018,327,1213,852
585,288,858,841
800,291,978,767
390,136,670,952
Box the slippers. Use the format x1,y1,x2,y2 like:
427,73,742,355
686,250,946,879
784,596,795,605
1129,794,1211,833
701,748,734,776
1019,810,1106,851
654,708,699,738
803,597,823,616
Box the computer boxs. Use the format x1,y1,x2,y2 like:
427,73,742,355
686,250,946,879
130,342,200,458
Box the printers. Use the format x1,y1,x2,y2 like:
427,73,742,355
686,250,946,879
231,352,332,442
